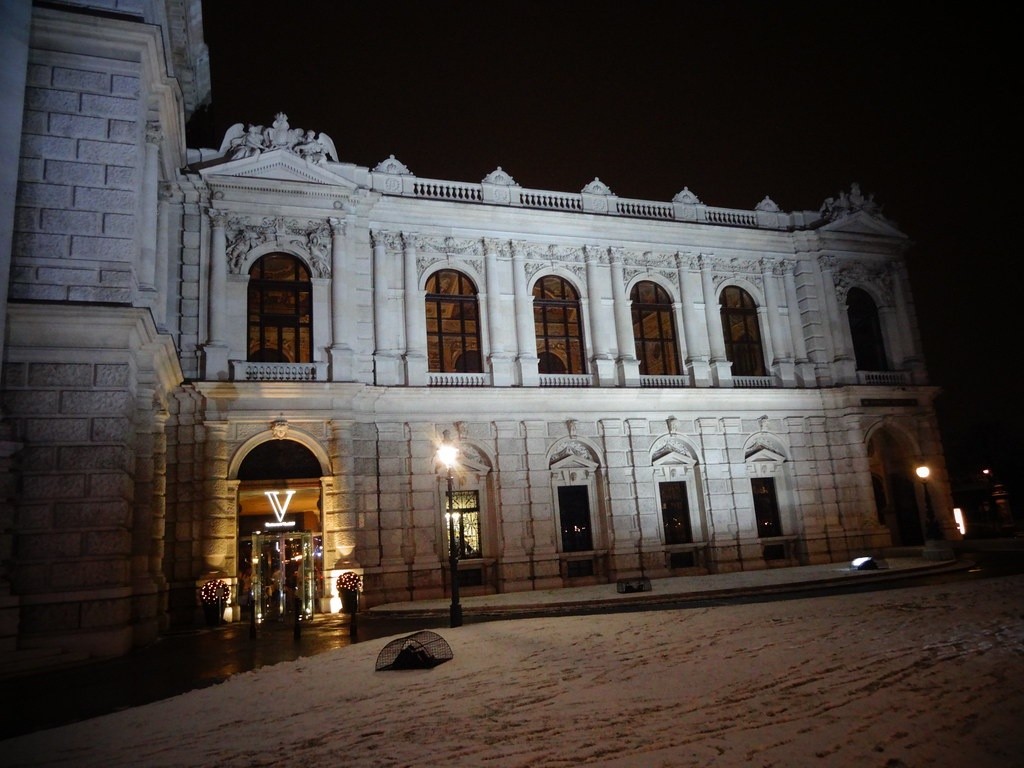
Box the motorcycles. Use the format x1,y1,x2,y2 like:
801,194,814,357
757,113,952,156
979,466,1017,540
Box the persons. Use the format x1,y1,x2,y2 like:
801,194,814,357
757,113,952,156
229,125,268,160
294,130,327,166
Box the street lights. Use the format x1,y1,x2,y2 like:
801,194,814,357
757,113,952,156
917,464,947,543
435,444,464,609
